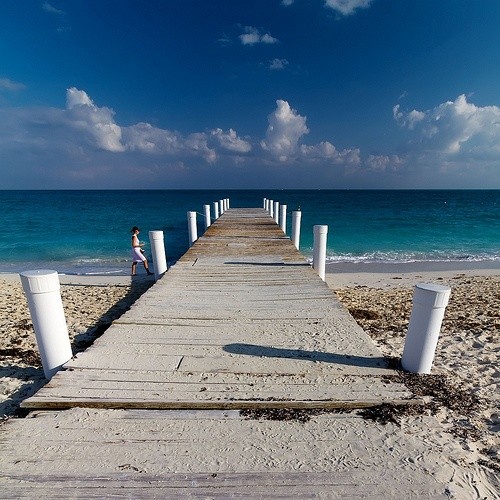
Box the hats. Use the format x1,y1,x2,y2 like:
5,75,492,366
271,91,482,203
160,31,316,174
133,226,139,230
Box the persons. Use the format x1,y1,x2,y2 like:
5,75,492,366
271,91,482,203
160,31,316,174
130,226,155,276
